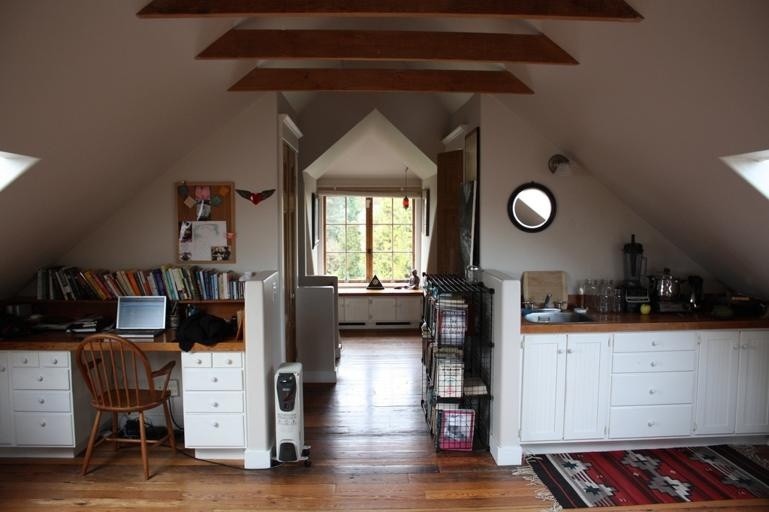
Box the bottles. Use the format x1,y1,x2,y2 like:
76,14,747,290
578,278,622,313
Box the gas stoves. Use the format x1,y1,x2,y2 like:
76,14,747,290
654,298,690,312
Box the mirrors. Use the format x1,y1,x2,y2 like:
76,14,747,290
506,180,555,233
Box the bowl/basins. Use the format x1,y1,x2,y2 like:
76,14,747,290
4,304,34,318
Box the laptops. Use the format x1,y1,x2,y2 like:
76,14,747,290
105,295,168,339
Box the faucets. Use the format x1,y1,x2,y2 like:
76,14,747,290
542,292,553,307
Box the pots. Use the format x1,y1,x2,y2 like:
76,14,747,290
646,268,688,301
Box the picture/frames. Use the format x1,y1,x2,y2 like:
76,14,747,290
312,191,322,248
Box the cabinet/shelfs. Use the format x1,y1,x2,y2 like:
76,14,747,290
516,331,605,441
339,297,369,323
370,295,421,323
0,349,72,448
181,352,245,448
420,271,495,457
610,333,692,437
19,265,245,332
692,332,769,434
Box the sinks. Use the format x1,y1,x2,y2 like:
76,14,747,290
527,311,593,323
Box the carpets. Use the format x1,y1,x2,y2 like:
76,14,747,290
510,442,769,512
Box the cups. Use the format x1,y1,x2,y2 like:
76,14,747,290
168,314,182,331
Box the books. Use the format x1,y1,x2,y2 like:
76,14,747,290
7,263,247,334
420,278,476,453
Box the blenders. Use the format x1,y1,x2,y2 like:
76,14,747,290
621,234,650,314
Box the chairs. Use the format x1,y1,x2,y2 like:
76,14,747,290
74,333,176,481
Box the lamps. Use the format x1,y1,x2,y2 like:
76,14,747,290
401,172,411,210
